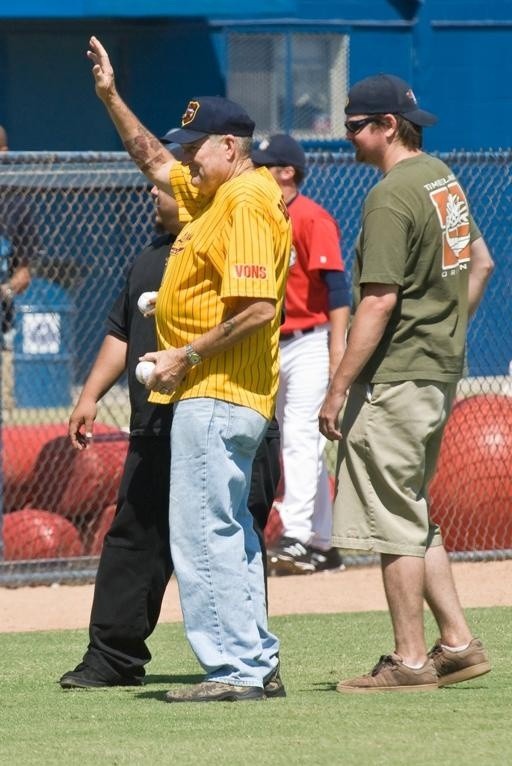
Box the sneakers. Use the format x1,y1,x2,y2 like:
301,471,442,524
334,651,440,693
164,668,286,700
425,636,491,687
61,663,145,688
266,535,341,576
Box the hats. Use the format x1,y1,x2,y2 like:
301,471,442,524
159,96,254,146
343,74,439,128
247,133,310,177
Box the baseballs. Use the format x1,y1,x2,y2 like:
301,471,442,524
137,292,157,314
135,360,155,384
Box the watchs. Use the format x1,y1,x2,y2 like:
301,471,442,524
186,347,202,366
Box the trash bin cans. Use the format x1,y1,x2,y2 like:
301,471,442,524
12,278,75,407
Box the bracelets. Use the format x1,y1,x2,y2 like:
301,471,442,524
7,282,14,297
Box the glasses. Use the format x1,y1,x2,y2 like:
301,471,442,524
344,118,385,135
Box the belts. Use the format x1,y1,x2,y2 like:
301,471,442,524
279,327,315,342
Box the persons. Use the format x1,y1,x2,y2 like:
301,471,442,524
86,37,291,701
251,134,351,577
317,75,492,694
59,128,185,688
0,125,40,422
